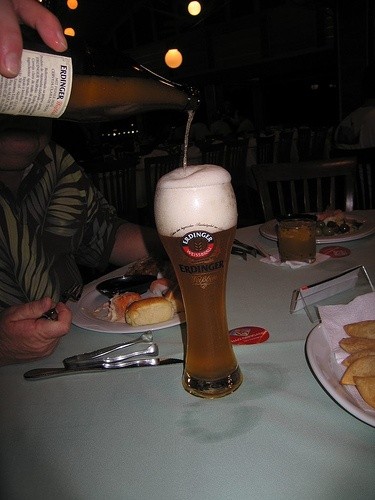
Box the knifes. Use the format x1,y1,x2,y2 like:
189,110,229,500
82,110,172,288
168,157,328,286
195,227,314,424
23,357,184,381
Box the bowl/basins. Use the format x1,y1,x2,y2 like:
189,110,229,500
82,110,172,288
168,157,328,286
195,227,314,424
95,274,157,300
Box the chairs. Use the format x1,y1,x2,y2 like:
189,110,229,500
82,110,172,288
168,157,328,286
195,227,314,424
89,125,375,225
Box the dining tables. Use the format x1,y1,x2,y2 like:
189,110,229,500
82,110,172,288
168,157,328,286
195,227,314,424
0,212,375,500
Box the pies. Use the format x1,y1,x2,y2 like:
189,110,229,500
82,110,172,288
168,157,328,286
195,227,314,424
339,319,375,407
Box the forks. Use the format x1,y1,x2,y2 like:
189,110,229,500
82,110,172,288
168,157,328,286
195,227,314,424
64,272,186,334
42,282,85,320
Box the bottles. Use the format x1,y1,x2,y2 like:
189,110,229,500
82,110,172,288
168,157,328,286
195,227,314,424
0,40,202,121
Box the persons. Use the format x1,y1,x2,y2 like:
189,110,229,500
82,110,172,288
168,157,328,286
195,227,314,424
191,110,253,136
0,0,67,78
324,65,375,158
0,114,163,367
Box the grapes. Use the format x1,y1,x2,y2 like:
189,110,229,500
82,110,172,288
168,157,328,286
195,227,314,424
315,221,349,236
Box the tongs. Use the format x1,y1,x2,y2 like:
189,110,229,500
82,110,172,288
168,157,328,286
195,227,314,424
62,328,160,371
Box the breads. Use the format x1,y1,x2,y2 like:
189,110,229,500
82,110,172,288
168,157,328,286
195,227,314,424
125,280,186,327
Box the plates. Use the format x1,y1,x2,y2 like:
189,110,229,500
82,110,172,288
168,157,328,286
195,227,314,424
259,212,375,243
305,322,375,427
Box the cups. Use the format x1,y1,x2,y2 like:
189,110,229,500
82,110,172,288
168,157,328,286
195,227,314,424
153,181,245,400
276,214,318,264
